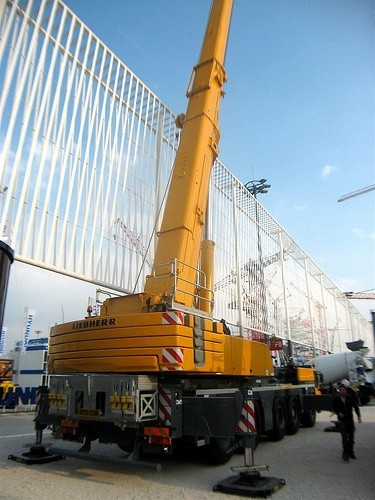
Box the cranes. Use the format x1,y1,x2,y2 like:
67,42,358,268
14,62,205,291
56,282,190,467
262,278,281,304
212,244,298,333
110,214,154,270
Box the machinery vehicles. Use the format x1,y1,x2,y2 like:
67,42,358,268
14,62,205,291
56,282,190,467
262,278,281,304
8,0,375,497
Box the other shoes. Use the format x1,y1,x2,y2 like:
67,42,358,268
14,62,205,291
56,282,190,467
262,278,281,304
343,456,349,460
350,455,356,459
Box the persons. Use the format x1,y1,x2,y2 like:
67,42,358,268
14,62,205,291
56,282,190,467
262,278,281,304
329,383,363,465
